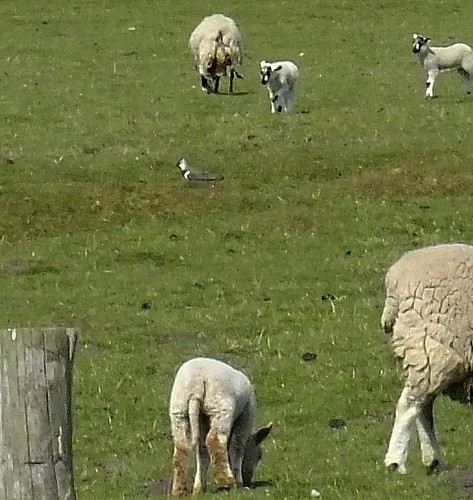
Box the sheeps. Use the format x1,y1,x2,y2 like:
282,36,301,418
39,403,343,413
168,357,272,495
381,243,472,474
187,13,244,93
259,59,299,112
411,32,473,99
